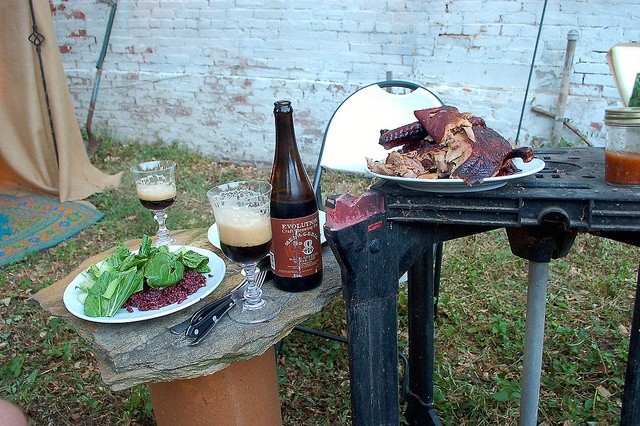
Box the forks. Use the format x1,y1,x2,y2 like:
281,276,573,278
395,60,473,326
185,270,267,347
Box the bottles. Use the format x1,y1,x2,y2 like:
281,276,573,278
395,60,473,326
269,99,323,293
603,105,635,189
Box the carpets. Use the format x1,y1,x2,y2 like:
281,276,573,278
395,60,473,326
1,193,106,271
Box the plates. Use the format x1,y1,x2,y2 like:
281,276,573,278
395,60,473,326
365,154,546,192
207,211,328,250
63,243,227,325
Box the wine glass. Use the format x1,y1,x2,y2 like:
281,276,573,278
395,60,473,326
205,179,281,325
129,160,179,248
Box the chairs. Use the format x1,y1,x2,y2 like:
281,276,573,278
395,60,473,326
279,79,452,405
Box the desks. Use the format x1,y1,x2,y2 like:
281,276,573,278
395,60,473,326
324,146,639,425
